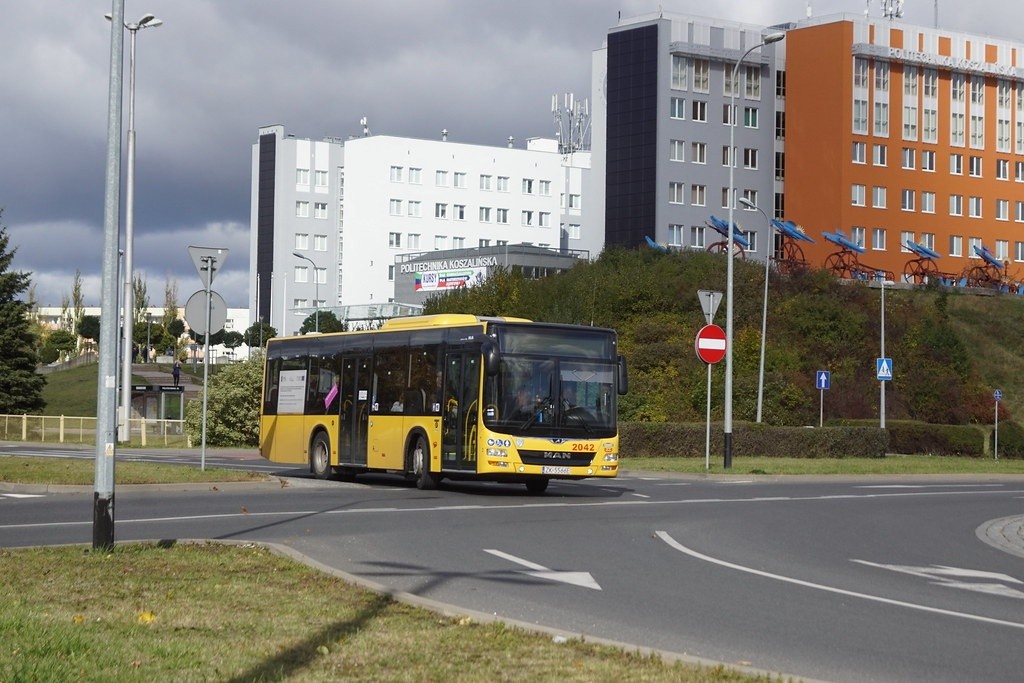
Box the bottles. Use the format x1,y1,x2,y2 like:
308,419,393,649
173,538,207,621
538,411,542,423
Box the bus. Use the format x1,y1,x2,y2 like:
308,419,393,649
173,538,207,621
258,314,629,490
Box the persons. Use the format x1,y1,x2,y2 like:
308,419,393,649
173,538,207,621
132,347,137,363
142,346,155,362
560,388,574,412
171,362,181,385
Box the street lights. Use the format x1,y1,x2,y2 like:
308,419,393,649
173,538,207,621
724,33,785,468
147,313,152,364
105,13,164,444
247,329,252,361
293,253,318,334
259,315,265,362
738,198,770,423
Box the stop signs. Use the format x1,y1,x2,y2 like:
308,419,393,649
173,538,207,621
695,325,728,364
